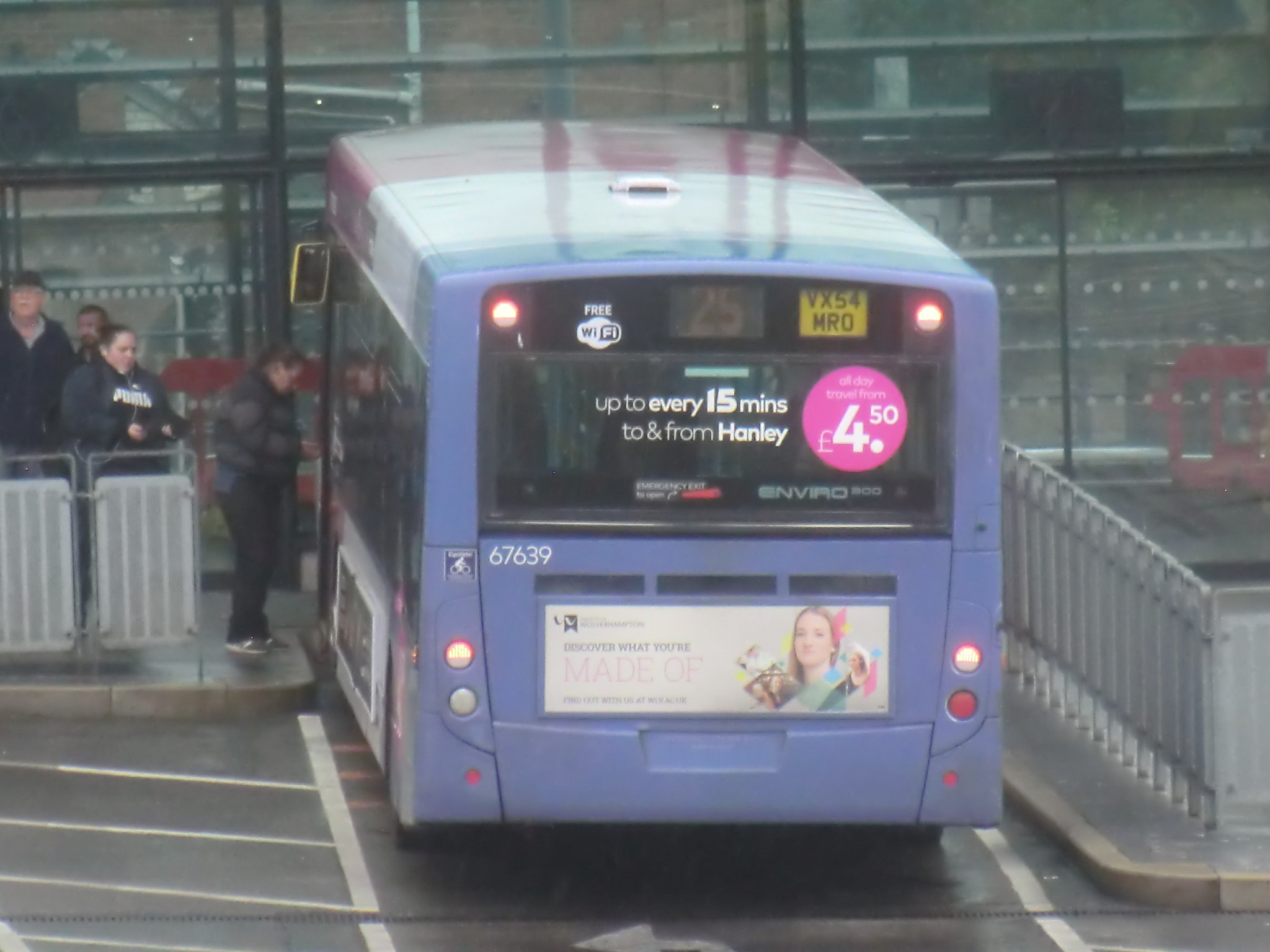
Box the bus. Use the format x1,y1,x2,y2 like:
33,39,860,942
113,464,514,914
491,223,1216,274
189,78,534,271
286,116,1006,863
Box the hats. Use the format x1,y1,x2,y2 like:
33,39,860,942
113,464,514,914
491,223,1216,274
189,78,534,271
9,272,48,291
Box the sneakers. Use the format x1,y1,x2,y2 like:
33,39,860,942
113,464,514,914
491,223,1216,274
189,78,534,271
257,635,290,651
226,638,273,655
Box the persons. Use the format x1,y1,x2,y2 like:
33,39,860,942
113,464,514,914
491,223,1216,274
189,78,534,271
208,340,321,657
747,606,870,711
0,271,188,637
